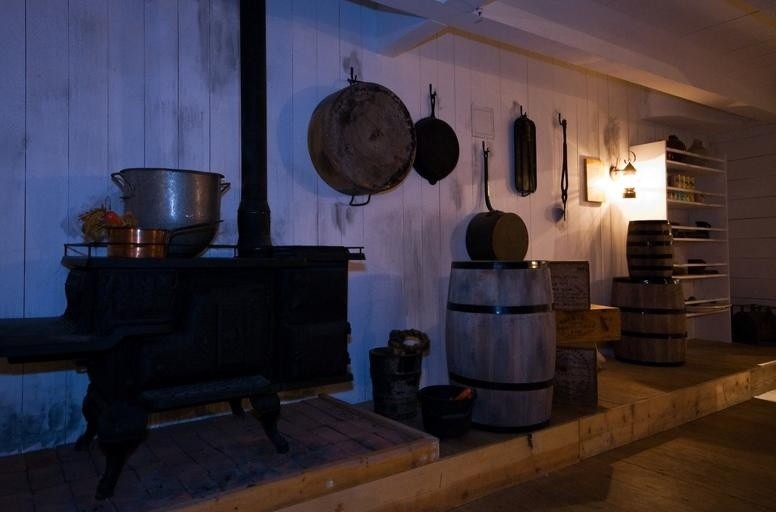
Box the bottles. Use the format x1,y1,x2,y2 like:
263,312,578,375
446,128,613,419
667,171,707,204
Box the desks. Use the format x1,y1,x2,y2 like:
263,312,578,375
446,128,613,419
58,241,369,494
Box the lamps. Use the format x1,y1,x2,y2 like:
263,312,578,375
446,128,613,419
609,146,638,201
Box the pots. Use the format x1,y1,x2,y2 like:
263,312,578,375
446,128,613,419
108,220,224,245
106,244,168,260
413,91,462,184
464,149,531,262
306,80,417,207
112,163,229,260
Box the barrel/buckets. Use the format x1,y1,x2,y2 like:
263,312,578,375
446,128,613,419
612,278,688,368
626,220,674,282
369,347,422,422
445,259,558,434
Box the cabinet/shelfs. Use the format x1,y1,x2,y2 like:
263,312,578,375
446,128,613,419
629,139,733,345
